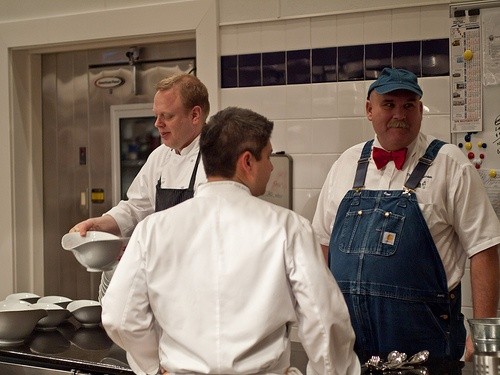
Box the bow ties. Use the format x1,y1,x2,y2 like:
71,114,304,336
372,147,408,171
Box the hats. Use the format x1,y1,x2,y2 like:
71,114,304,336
367,67,423,99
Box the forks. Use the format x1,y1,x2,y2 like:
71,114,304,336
361,355,380,375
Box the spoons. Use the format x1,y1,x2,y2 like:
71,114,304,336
382,350,430,370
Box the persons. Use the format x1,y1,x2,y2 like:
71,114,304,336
310,68,499,374
101,106,363,375
67,73,210,249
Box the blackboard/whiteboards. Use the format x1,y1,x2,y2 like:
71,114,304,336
255,153,294,211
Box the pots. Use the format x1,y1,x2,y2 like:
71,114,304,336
467,316,500,356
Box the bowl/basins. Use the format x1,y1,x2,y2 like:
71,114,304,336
0,302,48,346
6,292,41,304
30,303,66,331
67,300,102,326
37,296,72,309
62,231,129,271
